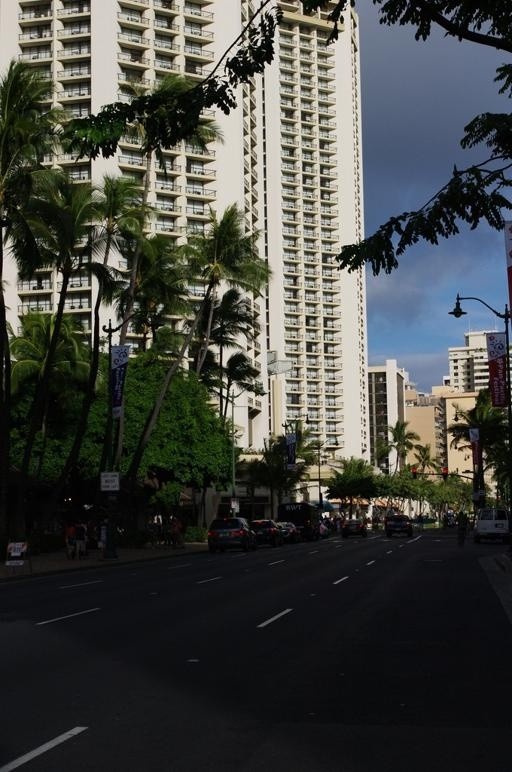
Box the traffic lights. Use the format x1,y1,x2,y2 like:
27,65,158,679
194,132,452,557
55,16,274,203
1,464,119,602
441,466,449,481
411,466,417,480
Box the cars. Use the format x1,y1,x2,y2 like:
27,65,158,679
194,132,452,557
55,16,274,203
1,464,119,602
340,518,368,540
249,518,284,548
278,521,298,544
207,516,256,552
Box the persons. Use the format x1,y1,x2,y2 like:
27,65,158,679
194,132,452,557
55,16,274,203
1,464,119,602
417,514,423,533
454,510,467,547
64,519,89,561
154,515,188,549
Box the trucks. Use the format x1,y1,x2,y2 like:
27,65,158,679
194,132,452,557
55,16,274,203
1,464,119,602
277,502,322,541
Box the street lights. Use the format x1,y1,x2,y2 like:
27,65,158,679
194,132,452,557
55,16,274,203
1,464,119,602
282,414,311,504
106,302,166,471
447,295,509,427
455,413,479,511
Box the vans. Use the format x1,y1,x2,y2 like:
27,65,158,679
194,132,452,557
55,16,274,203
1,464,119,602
384,514,413,538
472,507,509,544
